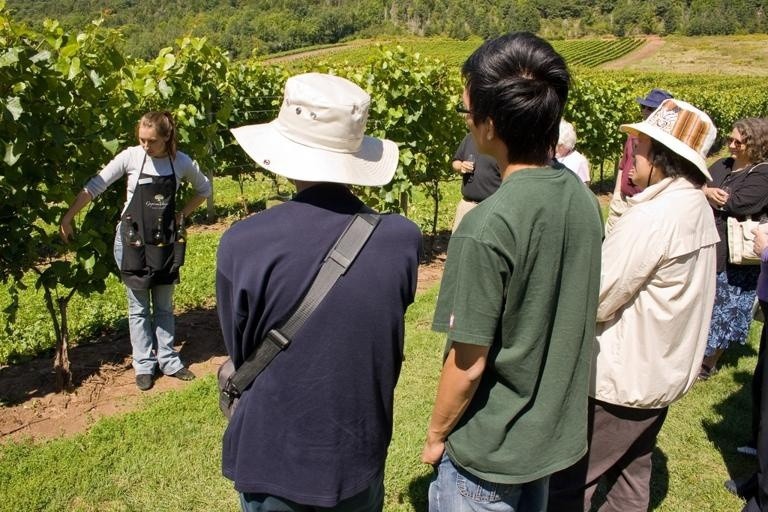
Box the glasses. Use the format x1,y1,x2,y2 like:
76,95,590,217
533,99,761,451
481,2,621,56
454,100,476,121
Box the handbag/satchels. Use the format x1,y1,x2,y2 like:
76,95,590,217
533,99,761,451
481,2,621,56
216,358,241,420
726,215,768,268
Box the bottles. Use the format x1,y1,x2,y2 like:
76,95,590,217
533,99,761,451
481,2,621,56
124,213,187,247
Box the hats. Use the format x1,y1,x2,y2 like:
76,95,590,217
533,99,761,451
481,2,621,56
620,98,717,183
634,88,677,110
230,72,400,187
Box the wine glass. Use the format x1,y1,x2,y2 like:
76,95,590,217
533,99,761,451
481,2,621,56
716,184,731,212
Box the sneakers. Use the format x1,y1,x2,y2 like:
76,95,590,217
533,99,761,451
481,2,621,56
135,373,153,390
173,367,195,381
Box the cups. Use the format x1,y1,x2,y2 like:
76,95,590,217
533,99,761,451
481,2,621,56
468,153,476,173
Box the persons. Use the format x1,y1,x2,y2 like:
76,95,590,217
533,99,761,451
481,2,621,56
216,73,423,511
448,132,502,235
60,111,213,390
702,117,768,380
605,90,674,238
725,225,768,512
550,99,721,511
420,32,606,511
555,119,590,187
